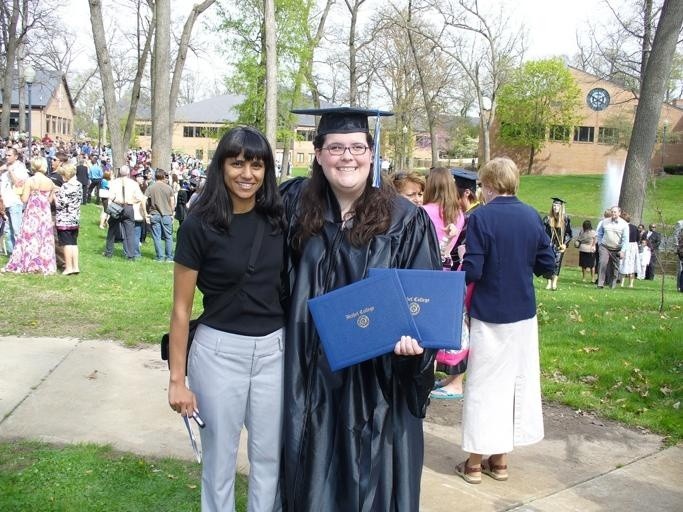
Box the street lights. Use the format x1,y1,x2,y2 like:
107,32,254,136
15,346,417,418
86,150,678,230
23,57,35,177
401,125,407,168
97,98,102,155
656,120,668,176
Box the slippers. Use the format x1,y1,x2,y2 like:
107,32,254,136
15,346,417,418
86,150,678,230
430,387,464,399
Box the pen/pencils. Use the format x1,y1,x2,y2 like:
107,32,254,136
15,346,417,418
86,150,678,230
185,408,205,428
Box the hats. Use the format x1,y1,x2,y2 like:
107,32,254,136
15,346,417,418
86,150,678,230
288,107,393,188
449,168,480,191
552,198,567,206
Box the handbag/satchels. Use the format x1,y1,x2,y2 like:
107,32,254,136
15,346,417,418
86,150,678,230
105,201,124,220
161,320,198,376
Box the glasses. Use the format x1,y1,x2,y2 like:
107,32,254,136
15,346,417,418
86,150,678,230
319,142,369,155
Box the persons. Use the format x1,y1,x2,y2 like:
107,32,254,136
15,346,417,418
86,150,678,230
275,160,290,178
279,106,443,511
454,156,557,485
390,172,485,396
578,206,661,289
673,220,683,292
543,197,572,290
168,125,287,511
0,133,208,275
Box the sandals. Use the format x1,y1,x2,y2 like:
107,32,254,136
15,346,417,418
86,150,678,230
454,458,482,484
481,455,508,481
